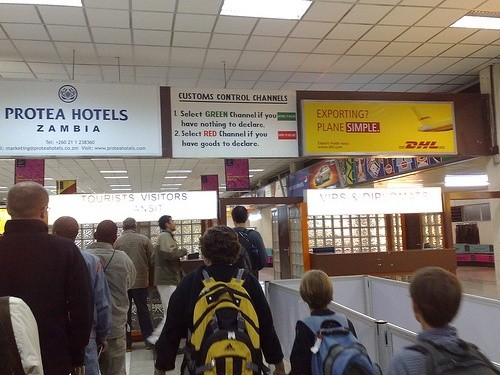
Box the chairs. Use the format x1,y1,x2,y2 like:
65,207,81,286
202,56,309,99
313,247,335,253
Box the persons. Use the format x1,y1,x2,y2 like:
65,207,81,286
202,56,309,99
289,269,360,374
51,216,111,375
0,296,43,375
1,182,95,375
385,266,499,373
82,219,137,375
113,217,155,352
231,205,268,279
145,215,187,349
152,225,285,375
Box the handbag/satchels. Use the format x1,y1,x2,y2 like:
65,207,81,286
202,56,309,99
240,230,267,271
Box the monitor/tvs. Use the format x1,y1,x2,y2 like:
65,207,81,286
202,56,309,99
313,246,335,253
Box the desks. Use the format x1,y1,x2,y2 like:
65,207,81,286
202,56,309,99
180,257,204,274
309,248,457,277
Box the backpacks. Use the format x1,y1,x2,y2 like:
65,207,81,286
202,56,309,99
410,339,500,375
187,265,269,375
298,312,377,375
